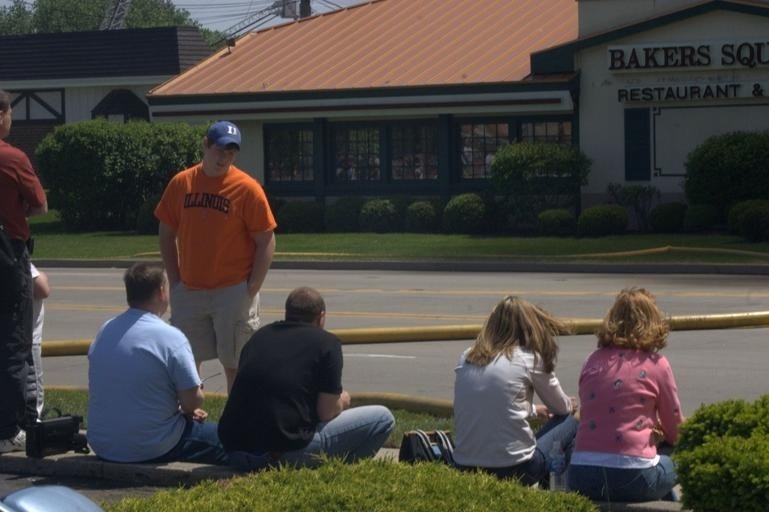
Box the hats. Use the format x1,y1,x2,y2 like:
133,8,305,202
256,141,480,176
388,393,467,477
208,121,242,151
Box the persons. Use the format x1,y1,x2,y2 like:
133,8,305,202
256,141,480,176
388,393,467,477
217,285,395,472
562,285,687,502
0,90,50,452
27,256,51,427
153,119,277,408
85,264,233,468
451,293,579,494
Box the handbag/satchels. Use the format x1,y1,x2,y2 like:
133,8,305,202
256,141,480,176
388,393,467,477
399,430,454,465
26,415,79,458
